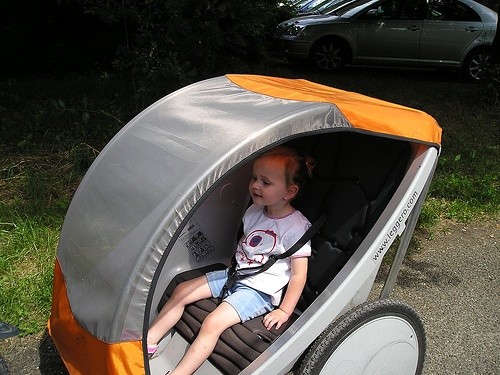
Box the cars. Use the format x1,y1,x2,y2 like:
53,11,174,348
275,0,498,81
278,0,379,16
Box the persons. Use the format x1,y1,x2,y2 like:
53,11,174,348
120,145,317,375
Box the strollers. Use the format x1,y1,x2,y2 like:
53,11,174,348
47,73,443,374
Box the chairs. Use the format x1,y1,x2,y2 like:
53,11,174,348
158,131,414,375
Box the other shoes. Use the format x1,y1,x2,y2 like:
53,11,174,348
121,330,159,358
